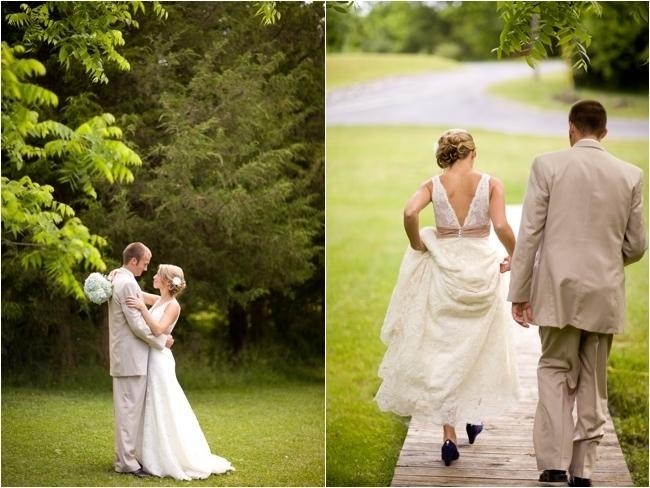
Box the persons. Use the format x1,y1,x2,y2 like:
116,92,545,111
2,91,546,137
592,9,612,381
374,129,515,466
506,99,645,486
108,241,176,477
107,263,237,480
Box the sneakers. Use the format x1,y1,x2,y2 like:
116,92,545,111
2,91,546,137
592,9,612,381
132,468,151,478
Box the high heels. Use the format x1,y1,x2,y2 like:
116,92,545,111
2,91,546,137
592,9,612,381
465,422,483,444
441,439,460,466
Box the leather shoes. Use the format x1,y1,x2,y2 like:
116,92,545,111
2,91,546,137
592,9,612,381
567,476,592,486
538,470,568,487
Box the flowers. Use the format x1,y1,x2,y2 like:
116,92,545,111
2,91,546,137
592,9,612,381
172,275,181,286
83,272,114,305
